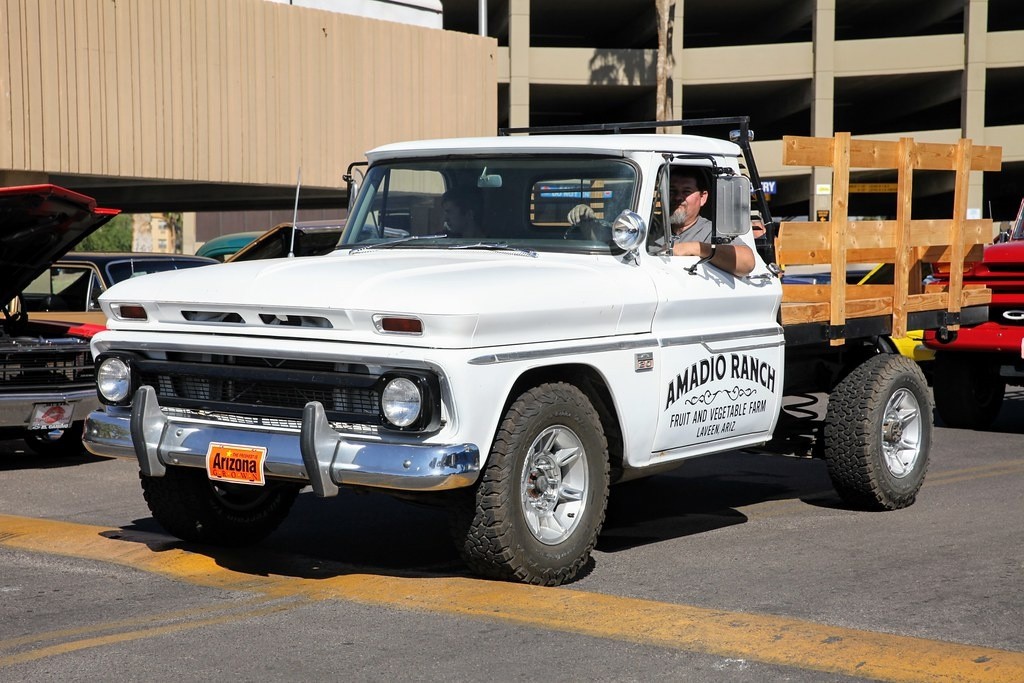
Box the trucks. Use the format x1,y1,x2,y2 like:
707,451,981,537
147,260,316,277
83,116,1006,587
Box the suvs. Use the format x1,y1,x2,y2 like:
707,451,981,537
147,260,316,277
921,188,1024,419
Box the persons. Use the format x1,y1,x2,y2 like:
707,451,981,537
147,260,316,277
567,166,754,277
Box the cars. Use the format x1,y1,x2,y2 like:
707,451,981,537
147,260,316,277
0,185,375,467
